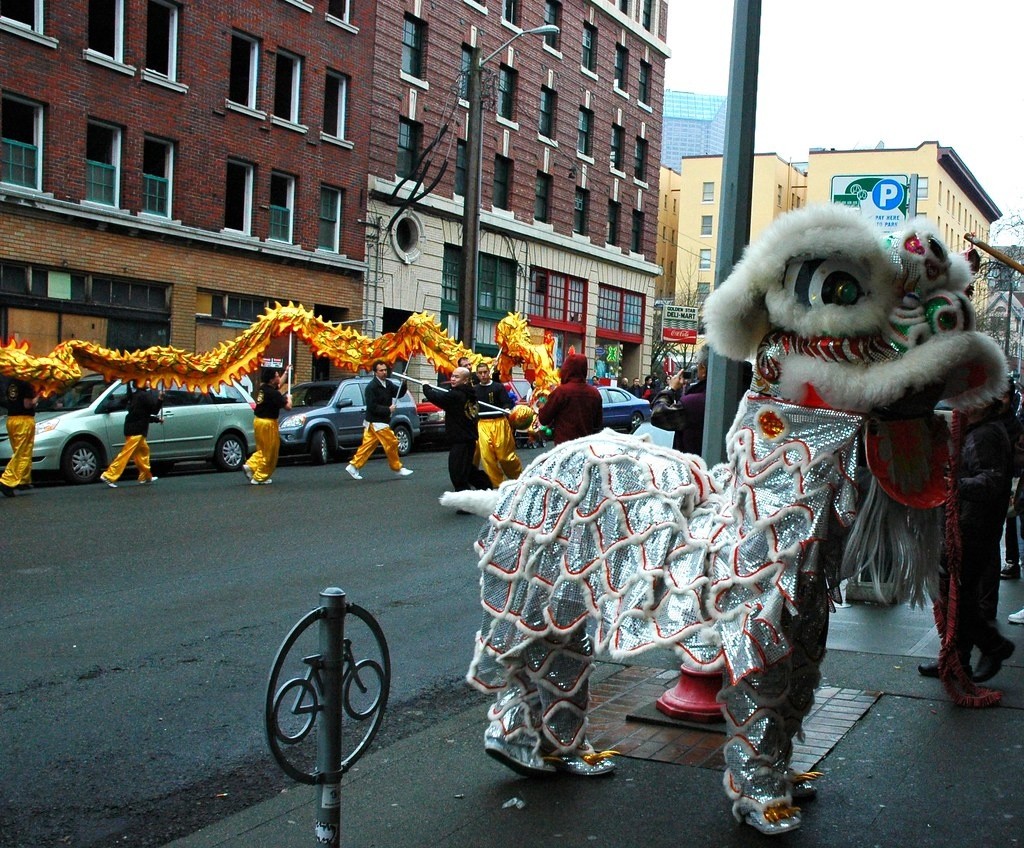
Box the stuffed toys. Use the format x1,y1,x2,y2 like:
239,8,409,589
438,202,1008,833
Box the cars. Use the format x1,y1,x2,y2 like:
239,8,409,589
596,386,652,433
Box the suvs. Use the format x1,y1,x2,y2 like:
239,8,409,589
277,377,420,466
414,379,535,441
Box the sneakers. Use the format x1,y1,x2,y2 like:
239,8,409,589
345,464,363,480
250,478,273,485
100,475,118,488
395,468,413,476
243,464,252,480
139,477,158,483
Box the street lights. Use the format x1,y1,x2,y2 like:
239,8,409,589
459,26,562,348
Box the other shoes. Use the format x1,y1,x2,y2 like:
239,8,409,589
18,485,34,490
918,660,940,675
1008,608,1024,623
971,638,1015,683
1000,562,1020,578
0,482,15,497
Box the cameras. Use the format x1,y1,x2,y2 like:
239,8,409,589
681,372,693,380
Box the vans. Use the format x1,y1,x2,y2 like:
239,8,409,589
0,371,258,485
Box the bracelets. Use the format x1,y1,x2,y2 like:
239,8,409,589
285,371,288,374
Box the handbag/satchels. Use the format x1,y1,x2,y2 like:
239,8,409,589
1005,477,1024,518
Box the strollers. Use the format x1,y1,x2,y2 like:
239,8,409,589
514,426,547,449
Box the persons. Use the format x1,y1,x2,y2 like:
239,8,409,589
651,342,754,458
918,370,1024,682
243,365,293,485
100,379,166,488
0,377,42,497
422,354,604,515
345,360,413,479
619,371,673,403
